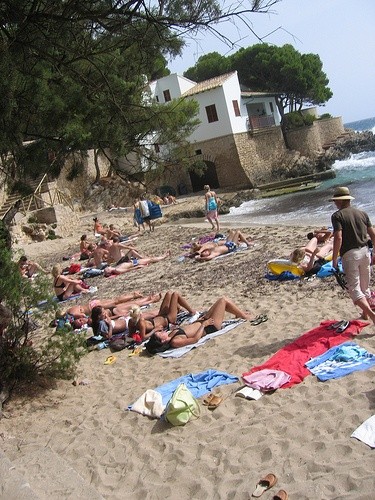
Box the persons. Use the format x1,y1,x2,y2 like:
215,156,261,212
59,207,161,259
292,226,375,279
147,297,249,354
328,187,375,325
12,181,263,346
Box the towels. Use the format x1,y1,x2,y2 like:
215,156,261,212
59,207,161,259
305,340,375,382
135,310,243,358
242,318,370,389
350,415,375,448
127,369,239,422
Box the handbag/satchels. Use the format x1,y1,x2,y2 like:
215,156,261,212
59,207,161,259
163,382,202,426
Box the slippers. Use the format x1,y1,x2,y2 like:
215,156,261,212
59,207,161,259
272,490,288,500
202,392,224,409
325,319,350,333
250,314,268,326
252,473,277,497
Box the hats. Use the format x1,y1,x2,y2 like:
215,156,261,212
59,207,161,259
328,187,356,202
